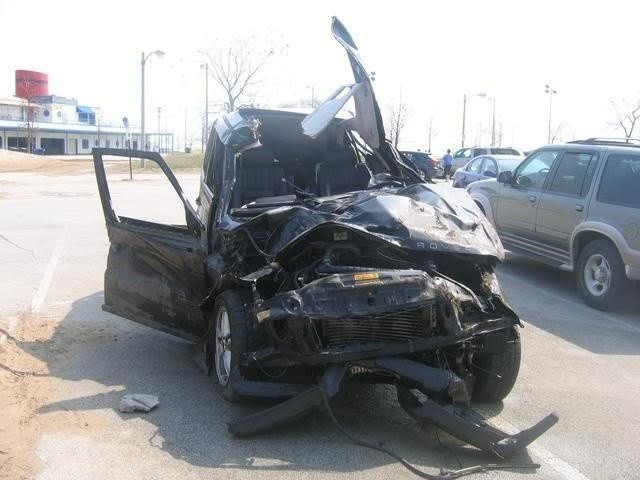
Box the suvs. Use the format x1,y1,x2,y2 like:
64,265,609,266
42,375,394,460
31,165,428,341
398,150,443,182
466,135,640,311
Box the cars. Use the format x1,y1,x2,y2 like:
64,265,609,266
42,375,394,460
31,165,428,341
451,155,551,190
90,16,559,480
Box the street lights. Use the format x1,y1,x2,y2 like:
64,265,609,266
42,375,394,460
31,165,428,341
139,47,165,169
461,92,489,150
198,61,209,146
543,82,558,145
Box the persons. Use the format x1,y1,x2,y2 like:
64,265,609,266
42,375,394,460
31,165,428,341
442,149,453,182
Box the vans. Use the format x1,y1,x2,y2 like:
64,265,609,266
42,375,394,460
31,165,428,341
439,146,523,178
